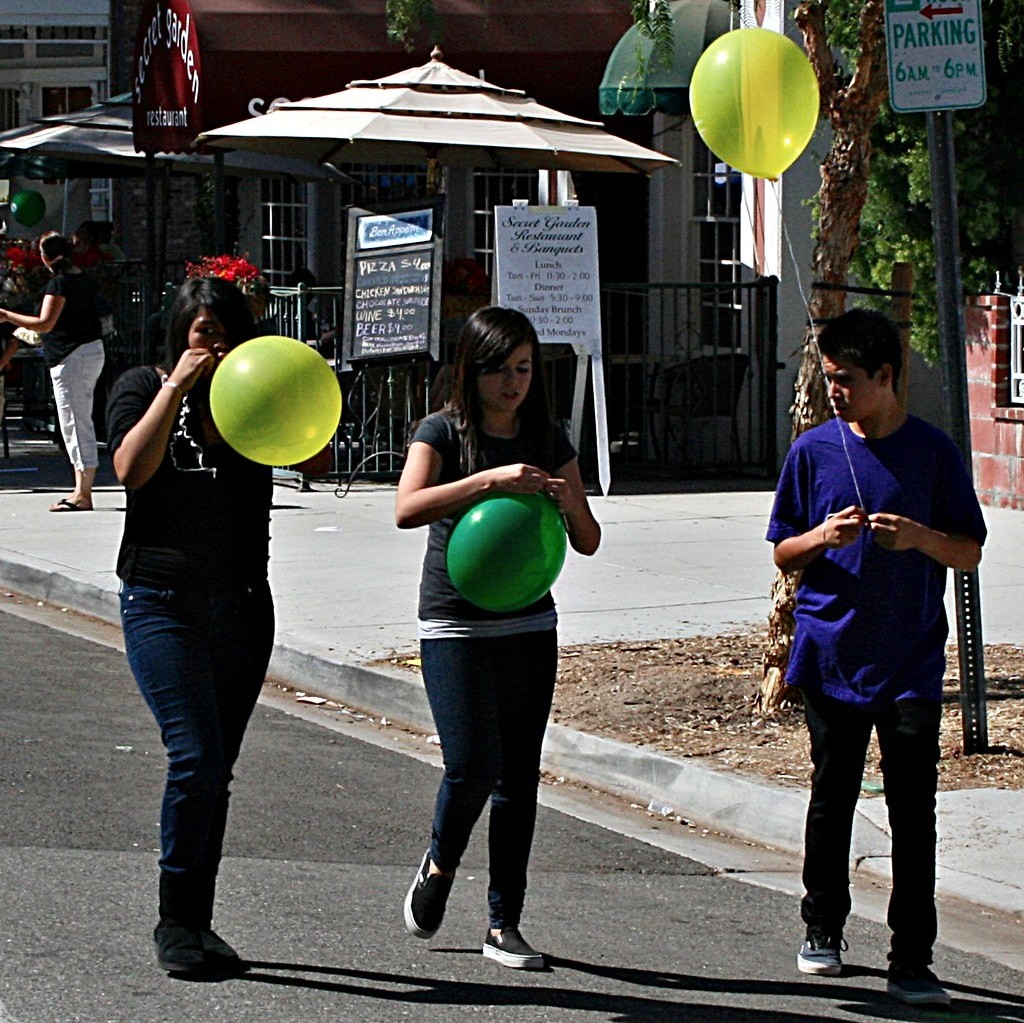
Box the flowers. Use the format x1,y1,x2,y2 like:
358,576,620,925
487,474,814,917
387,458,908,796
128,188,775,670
0,237,116,315
182,245,260,294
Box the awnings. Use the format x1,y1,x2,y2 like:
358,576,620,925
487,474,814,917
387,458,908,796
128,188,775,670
597,0,742,117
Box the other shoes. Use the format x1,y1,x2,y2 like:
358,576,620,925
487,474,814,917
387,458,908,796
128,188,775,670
482,924,544,969
402,846,456,939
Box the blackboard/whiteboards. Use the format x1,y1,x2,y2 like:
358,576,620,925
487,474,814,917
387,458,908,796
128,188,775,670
494,205,602,357
349,248,433,359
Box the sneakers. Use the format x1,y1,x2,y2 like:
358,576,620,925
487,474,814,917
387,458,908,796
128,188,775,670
796,933,848,976
886,964,953,1006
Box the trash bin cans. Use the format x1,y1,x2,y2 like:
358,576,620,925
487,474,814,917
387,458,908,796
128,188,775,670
107,346,139,391
12,346,57,437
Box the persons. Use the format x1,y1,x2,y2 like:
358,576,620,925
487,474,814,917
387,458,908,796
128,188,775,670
103,273,275,981
768,307,987,1008
394,307,601,970
0,219,115,511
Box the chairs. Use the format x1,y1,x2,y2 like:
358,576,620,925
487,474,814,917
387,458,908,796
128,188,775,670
640,355,747,477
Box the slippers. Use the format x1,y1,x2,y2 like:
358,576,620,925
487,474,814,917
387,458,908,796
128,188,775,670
51,497,94,511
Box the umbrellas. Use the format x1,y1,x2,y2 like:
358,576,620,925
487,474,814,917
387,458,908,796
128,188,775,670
1,94,355,235
184,46,684,209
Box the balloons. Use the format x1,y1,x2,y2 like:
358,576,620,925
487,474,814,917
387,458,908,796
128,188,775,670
444,489,567,612
690,29,821,179
9,190,46,228
210,334,342,468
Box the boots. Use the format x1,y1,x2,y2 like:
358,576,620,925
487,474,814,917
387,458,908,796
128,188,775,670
154,869,206,973
198,874,244,974
